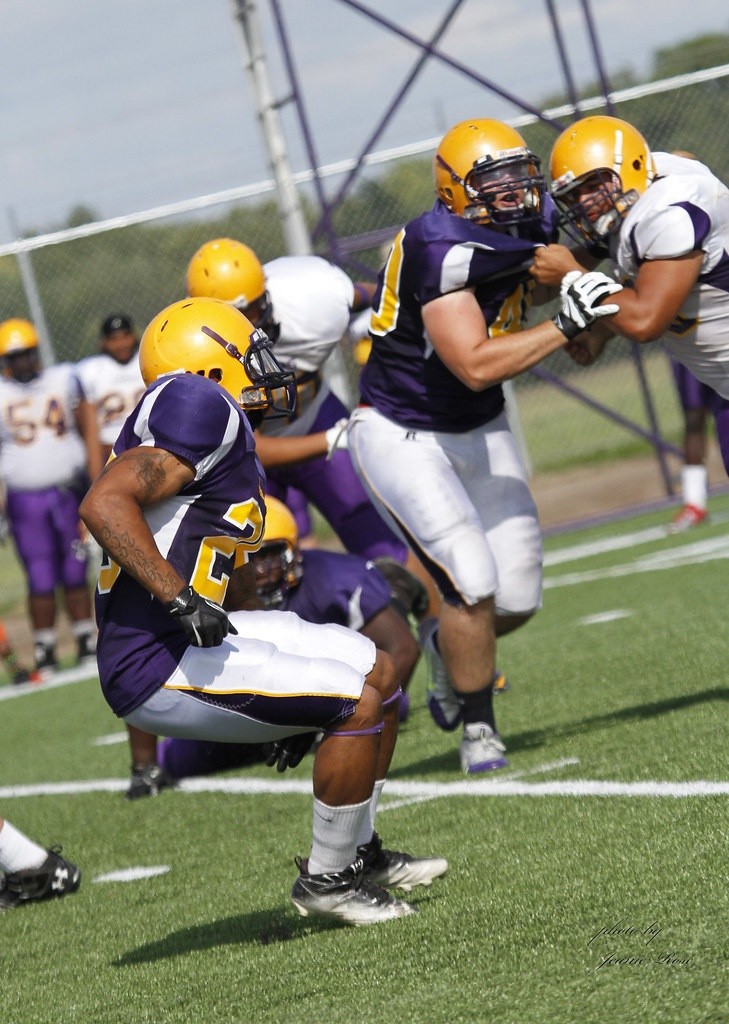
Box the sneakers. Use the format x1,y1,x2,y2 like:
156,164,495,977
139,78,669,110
459,721,507,774
0,846,82,909
415,619,462,733
291,831,447,929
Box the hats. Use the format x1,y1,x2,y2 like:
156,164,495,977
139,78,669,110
100,313,132,335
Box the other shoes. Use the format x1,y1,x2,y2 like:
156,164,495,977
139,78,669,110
665,505,709,537
73,631,97,665
30,640,60,681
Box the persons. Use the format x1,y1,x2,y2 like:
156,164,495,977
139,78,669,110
553,115,728,536
347,115,624,777
0,314,139,685
74,236,448,929
0,817,81,907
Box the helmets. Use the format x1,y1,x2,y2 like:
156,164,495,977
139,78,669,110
250,493,300,610
139,297,298,430
437,119,548,227
551,115,654,254
187,237,281,345
0,318,43,383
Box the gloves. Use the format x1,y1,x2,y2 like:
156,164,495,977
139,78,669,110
126,762,174,800
263,732,319,773
167,585,238,650
551,269,623,342
